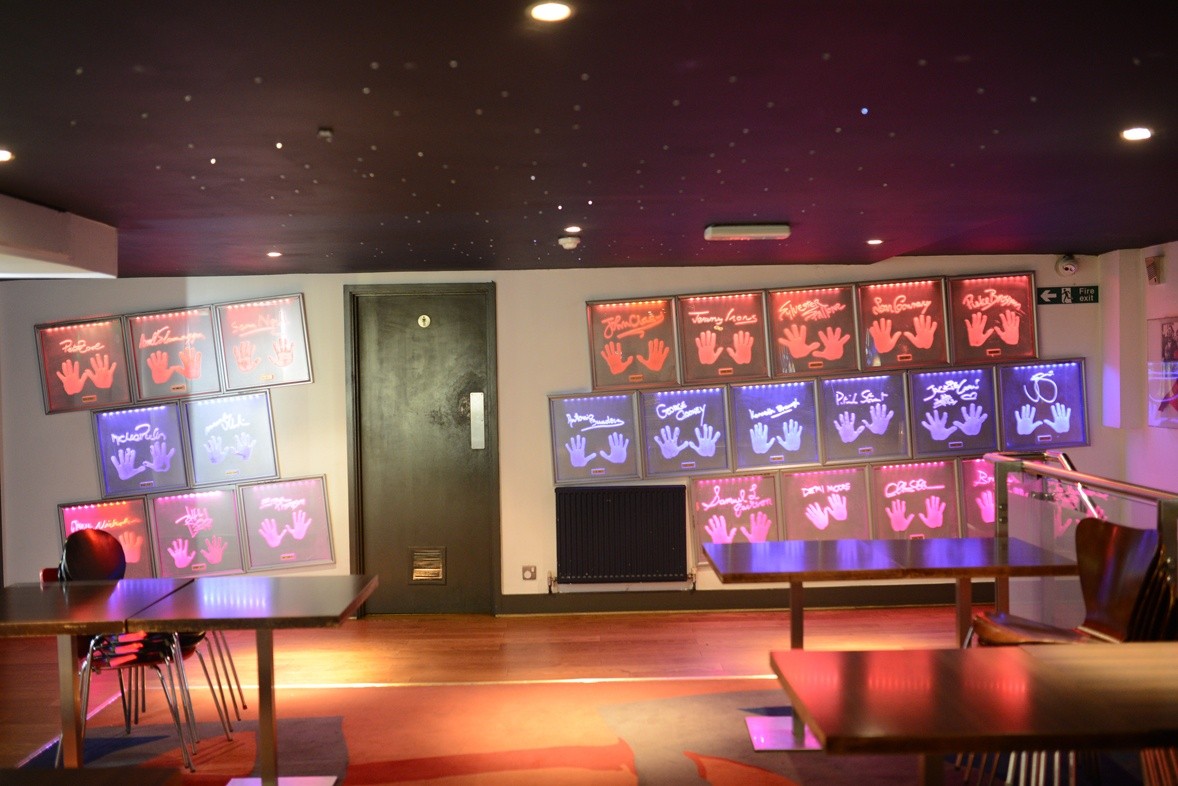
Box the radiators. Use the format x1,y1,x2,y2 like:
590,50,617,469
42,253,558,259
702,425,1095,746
547,485,697,594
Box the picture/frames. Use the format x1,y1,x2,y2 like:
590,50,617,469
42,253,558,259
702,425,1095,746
546,270,1093,566
33,290,338,580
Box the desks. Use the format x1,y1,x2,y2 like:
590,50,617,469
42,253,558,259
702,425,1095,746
862,535,1079,649
0,573,380,786
701,536,905,754
767,641,1178,786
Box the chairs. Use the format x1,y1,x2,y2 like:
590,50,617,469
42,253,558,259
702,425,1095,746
40,528,247,775
947,515,1178,786
